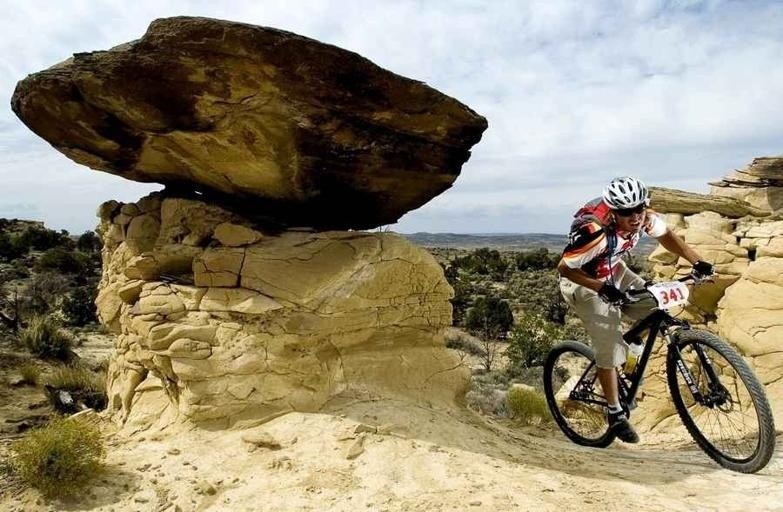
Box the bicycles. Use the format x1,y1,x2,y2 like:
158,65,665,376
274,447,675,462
542,256,780,478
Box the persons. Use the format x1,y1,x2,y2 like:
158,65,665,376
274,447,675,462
557,178,715,444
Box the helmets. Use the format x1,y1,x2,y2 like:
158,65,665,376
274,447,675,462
601,175,647,211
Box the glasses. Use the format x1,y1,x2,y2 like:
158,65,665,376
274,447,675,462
610,204,645,217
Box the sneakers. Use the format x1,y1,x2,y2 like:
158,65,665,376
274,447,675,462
607,410,639,443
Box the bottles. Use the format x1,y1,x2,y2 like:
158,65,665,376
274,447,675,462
624,335,645,375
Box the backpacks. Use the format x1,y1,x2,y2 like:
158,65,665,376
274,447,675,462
568,196,616,262
